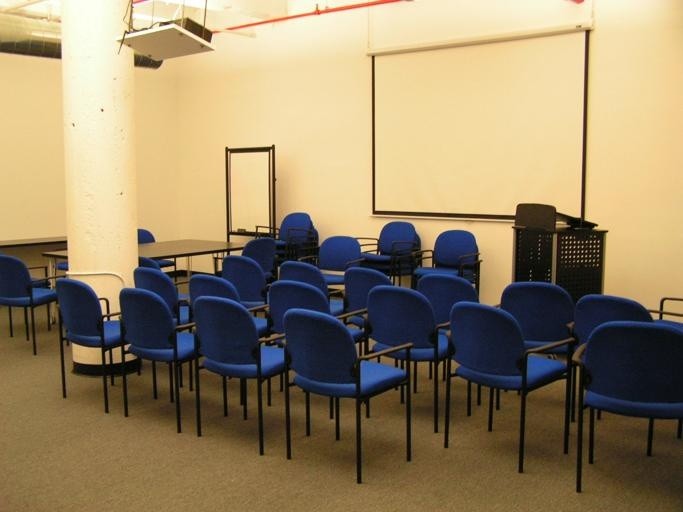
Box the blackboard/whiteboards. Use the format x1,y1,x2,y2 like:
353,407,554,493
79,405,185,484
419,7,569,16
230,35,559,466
225,144,276,239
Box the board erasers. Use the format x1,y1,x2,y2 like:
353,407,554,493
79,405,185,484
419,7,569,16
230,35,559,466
237,228,246,233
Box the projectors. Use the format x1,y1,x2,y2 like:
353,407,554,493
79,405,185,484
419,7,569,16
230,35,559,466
158,17,213,44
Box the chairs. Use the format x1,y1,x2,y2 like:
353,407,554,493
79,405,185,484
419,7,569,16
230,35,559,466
194,297,311,455
647,297,682,320
575,294,655,419
120,288,229,434
284,309,413,485
54,277,158,414
495,283,578,422
572,321,682,492
1,212,484,406
366,286,471,432
441,301,569,471
